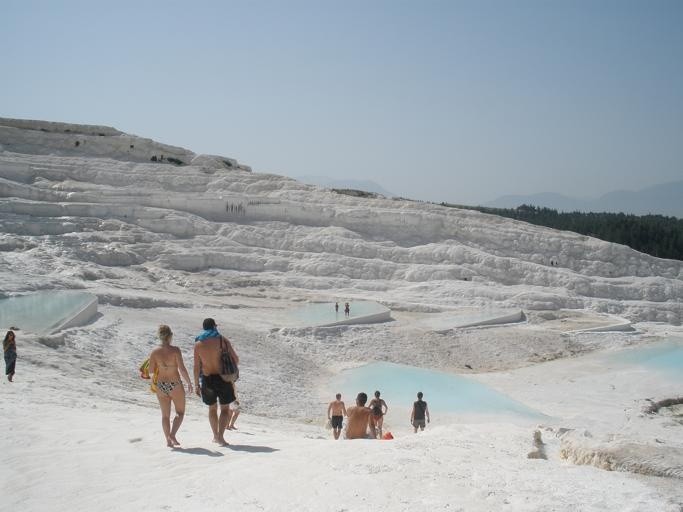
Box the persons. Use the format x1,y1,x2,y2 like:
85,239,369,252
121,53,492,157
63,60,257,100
367,390,389,439
341,391,377,441
223,381,241,431
334,302,337,312
193,317,239,447
409,391,429,432
1,329,16,382
343,301,350,316
149,323,193,450
326,392,347,440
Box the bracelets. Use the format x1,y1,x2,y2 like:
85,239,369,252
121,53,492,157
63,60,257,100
194,384,200,388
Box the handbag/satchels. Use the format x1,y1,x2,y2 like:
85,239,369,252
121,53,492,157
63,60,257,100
220,351,238,381
